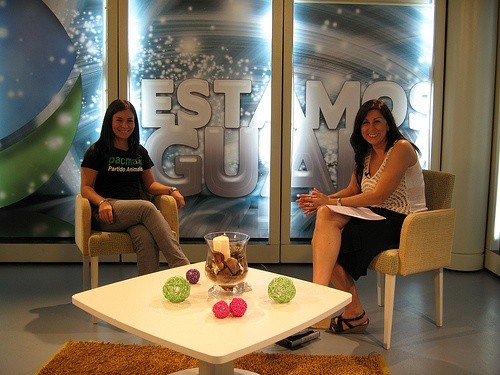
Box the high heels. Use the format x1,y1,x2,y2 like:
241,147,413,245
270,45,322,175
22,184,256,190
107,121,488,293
325,310,369,334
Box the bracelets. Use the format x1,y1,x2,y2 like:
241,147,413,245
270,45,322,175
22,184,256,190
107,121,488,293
97,198,109,207
336,197,342,206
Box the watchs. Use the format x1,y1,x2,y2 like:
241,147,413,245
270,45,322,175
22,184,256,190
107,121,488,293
168,187,178,195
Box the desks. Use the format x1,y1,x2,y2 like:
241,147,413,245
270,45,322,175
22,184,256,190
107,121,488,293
72,260,353,375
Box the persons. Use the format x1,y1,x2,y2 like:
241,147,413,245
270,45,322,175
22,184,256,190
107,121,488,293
79,99,190,278
295,99,429,334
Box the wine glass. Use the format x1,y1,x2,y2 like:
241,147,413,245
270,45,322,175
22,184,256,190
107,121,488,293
203,231,251,301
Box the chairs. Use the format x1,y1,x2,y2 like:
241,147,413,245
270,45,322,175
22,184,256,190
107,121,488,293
75,193,180,323
368,171,455,348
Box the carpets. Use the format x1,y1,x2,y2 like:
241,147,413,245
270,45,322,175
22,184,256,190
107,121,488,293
34,339,394,375
